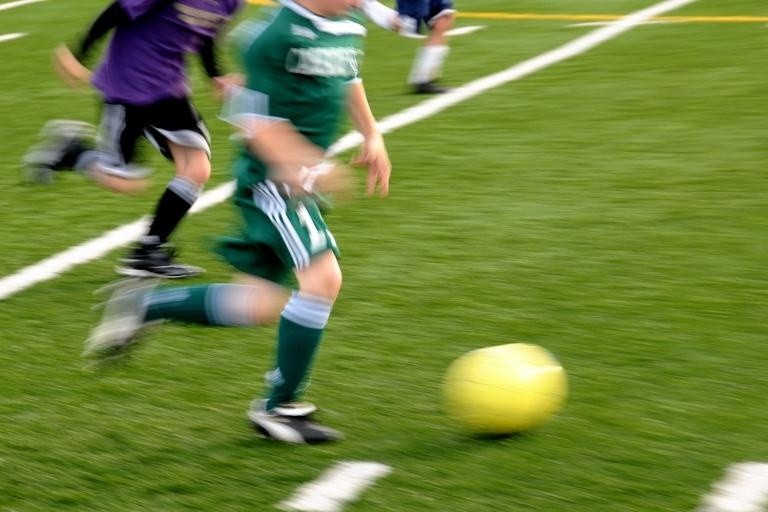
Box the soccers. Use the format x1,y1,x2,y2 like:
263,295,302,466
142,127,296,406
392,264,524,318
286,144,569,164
446,338,568,438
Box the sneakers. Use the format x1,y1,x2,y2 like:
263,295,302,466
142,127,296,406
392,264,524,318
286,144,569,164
25,134,97,172
85,286,146,358
112,252,207,278
410,82,449,93
246,401,345,444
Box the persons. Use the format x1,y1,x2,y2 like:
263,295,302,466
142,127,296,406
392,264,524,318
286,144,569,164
80,1,392,447
357,0,460,93
19,0,257,280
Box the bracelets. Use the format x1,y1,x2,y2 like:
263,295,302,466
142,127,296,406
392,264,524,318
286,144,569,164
293,158,337,191
